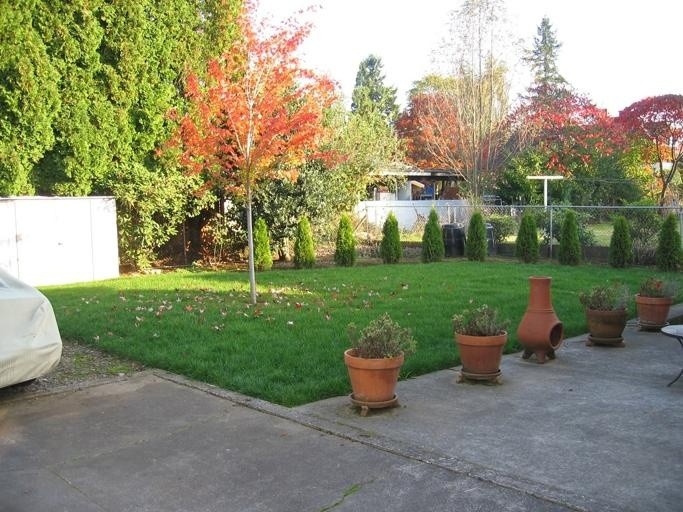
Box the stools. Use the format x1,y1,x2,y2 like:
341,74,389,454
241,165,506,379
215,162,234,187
660,325,683,387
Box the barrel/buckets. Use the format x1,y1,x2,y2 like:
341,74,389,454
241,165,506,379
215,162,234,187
484,223,497,256
442,223,464,257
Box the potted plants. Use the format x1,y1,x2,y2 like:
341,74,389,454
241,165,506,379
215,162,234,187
452,303,512,373
576,275,675,338
341,311,419,403
